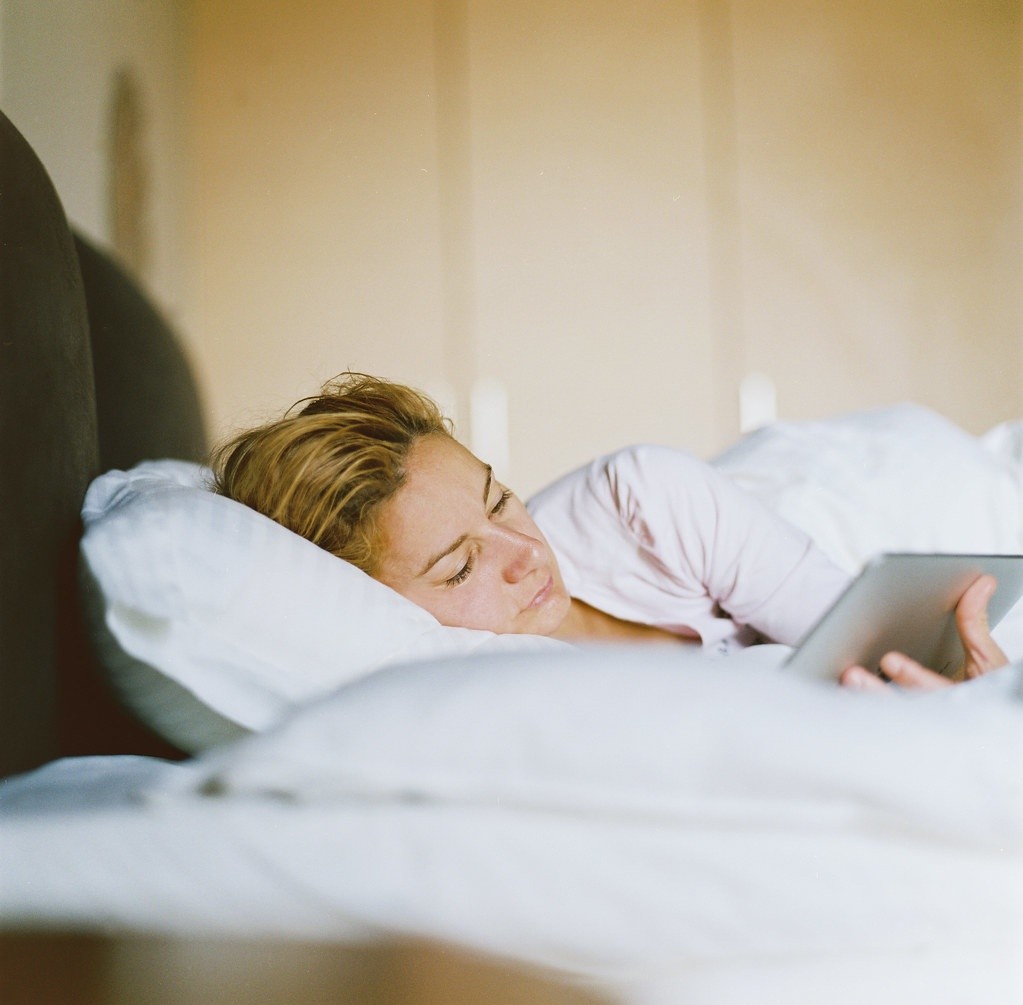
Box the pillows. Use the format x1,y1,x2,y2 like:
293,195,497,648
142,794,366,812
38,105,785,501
213,640,1023,857
80,455,567,763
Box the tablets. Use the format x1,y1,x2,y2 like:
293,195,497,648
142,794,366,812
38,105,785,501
779,551,1023,689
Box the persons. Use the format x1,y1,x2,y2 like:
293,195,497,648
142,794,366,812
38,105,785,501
213,373,1023,698
